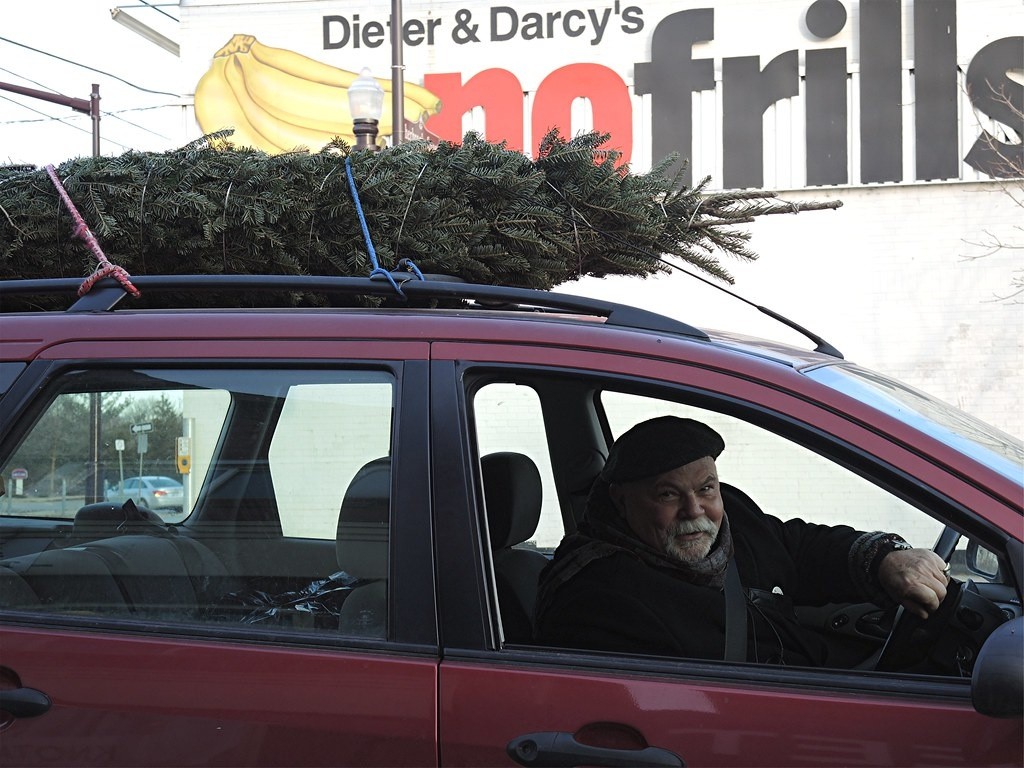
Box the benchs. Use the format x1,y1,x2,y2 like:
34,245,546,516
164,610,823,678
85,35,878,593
0,535,232,622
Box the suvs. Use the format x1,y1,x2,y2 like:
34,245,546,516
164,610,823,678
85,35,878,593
1,272,1024,767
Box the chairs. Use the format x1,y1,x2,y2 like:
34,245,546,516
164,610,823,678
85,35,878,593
480,451,551,645
335,456,392,639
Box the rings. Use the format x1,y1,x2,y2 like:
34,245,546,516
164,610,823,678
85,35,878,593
943,562,950,572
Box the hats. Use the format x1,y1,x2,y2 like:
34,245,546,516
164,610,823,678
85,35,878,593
599,415,725,483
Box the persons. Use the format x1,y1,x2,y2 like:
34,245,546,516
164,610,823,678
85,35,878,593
530,415,951,676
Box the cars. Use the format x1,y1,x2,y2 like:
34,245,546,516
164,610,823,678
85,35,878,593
107,475,185,515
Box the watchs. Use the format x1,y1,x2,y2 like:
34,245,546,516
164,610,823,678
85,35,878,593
871,540,912,583
